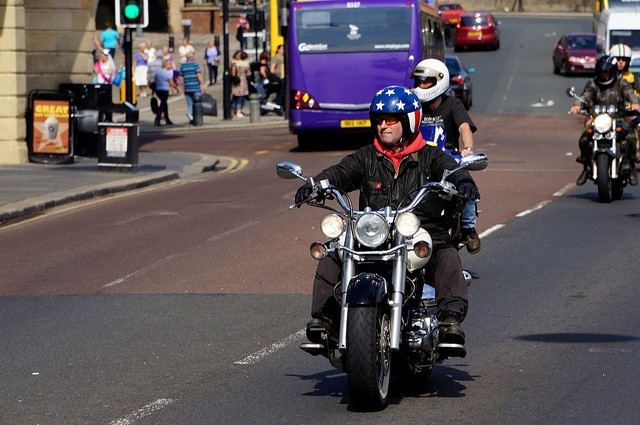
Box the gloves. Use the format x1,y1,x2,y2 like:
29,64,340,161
295,182,321,208
454,179,480,201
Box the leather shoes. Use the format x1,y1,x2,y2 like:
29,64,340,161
577,170,589,185
629,167,638,185
440,315,465,344
462,227,480,254
306,316,338,350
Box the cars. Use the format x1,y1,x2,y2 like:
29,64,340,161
444,56,477,110
438,3,467,26
552,32,604,72
453,11,502,50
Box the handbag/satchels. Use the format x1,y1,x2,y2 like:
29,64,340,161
201,93,217,116
230,63,240,86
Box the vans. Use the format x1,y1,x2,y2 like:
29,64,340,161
596,7,640,72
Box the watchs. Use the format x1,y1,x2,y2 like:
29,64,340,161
464,146,474,151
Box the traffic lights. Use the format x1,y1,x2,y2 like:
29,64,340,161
115,0,148,27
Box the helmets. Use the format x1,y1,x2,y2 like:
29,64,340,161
610,44,631,71
411,58,450,102
369,85,422,135
595,56,617,89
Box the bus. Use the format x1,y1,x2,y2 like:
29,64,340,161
280,0,451,147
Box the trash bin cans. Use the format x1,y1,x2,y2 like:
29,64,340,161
97,102,139,171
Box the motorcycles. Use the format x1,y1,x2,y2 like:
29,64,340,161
419,123,483,252
277,152,488,410
565,86,639,201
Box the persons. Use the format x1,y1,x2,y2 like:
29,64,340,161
93,49,116,85
236,13,249,52
230,51,250,118
259,51,281,110
410,58,481,255
295,85,478,346
179,38,197,63
146,41,176,68
270,45,285,79
582,44,640,165
113,54,136,105
179,52,206,120
570,56,637,186
204,41,222,86
100,22,120,58
151,59,179,125
133,43,148,99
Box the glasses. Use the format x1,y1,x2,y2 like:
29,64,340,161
375,116,399,125
